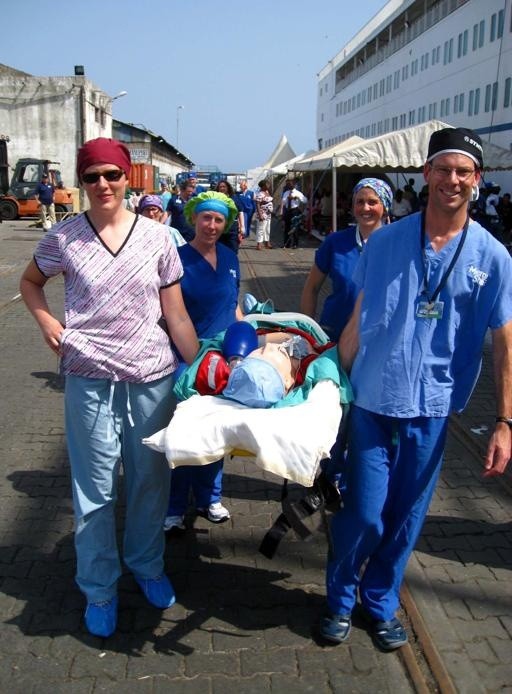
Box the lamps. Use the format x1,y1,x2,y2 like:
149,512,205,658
105,91,128,111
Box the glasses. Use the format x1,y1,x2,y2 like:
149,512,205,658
80,169,124,183
432,164,476,182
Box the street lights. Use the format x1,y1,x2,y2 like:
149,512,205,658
176,104,184,147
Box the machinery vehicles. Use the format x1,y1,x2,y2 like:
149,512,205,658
1,137,75,222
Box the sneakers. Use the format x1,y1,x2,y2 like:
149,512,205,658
163,515,186,532
134,573,176,608
198,502,230,523
377,618,408,649
322,608,352,642
85,596,118,637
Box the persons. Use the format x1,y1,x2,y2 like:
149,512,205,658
163,182,244,531
303,177,394,500
223,331,320,409
33,174,56,225
318,128,512,651
393,175,512,249
22,140,202,635
135,173,334,251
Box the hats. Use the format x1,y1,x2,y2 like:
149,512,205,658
427,128,483,167
139,172,238,234
354,178,393,215
224,358,284,409
77,138,131,186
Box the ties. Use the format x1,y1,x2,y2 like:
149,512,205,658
287,191,292,211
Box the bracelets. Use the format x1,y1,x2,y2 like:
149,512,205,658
494,416,512,428
